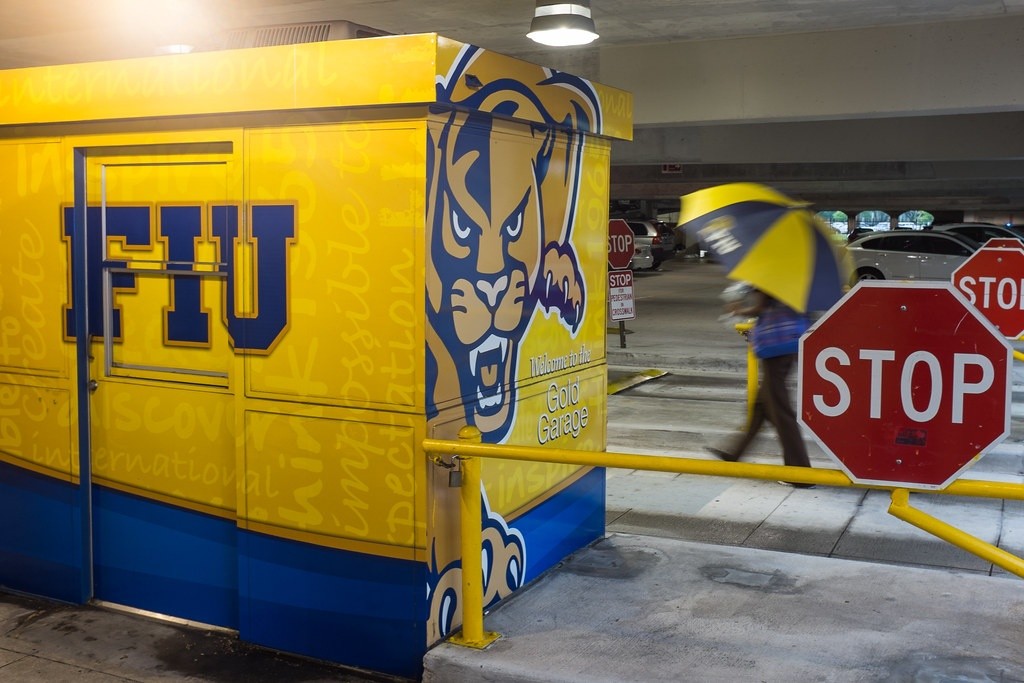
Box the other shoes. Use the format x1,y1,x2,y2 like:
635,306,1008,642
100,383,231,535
702,446,738,463
777,480,814,490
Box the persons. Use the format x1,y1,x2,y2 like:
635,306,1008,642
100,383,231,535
704,288,818,488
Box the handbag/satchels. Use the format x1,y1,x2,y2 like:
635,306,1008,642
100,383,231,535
750,310,811,358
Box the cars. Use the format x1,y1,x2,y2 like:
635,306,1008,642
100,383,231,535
624,218,678,272
832,220,1024,288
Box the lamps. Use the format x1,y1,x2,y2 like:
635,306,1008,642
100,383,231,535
524,0,600,48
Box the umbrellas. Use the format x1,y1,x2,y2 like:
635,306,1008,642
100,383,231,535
678,183,856,315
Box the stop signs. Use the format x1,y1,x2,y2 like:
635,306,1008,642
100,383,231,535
608,270,636,322
951,247,1024,340
607,219,635,269
794,278,1015,490
